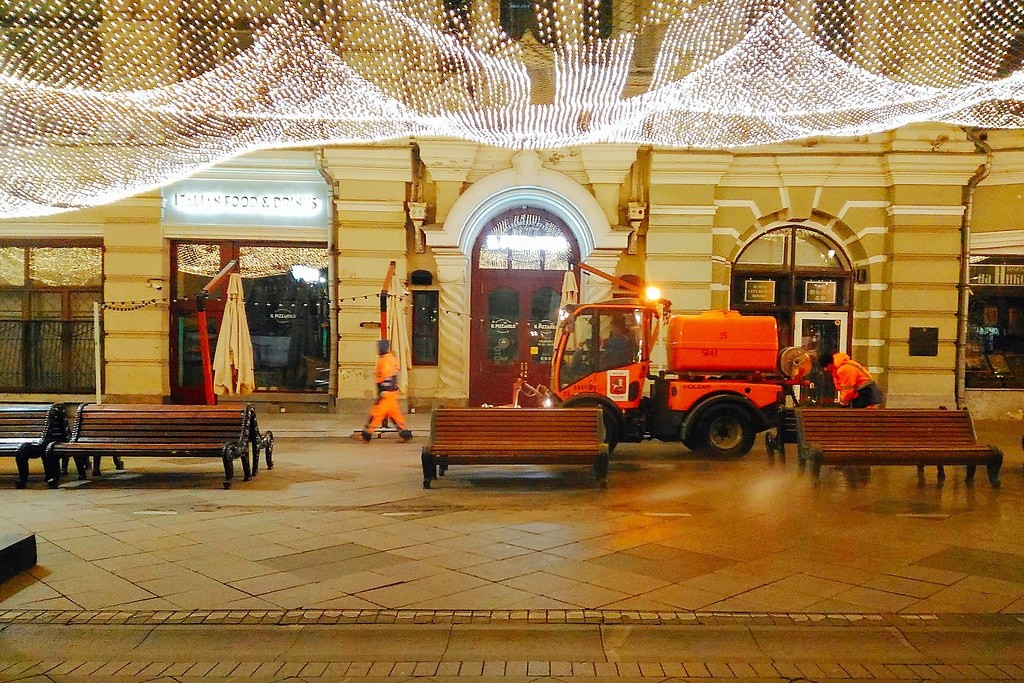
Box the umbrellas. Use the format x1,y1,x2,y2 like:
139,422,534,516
386,276,411,398
551,272,586,394
213,273,255,397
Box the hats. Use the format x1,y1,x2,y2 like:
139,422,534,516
610,313,632,332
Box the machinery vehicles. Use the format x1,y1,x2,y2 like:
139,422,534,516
540,254,818,458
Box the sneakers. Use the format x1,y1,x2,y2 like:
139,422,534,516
351,431,371,444
395,431,413,443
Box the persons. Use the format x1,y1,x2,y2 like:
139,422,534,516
355,340,414,443
815,351,884,409
595,312,636,370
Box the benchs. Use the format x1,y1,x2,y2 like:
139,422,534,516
766,407,1004,494
0,401,92,488
44,402,273,489
422,405,609,489
